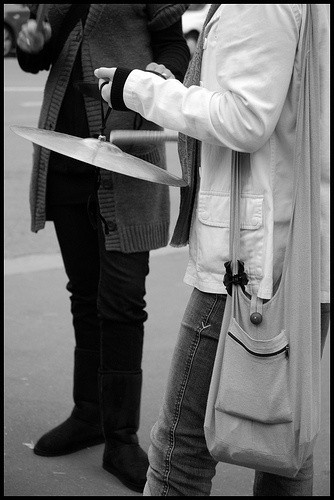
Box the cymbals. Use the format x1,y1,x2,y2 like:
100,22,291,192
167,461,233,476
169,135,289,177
8,124,188,188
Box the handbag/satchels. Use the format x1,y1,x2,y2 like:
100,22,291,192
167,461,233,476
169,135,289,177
203,222,322,479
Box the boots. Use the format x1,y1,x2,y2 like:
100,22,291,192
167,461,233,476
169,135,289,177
99,368,150,492
34,349,102,458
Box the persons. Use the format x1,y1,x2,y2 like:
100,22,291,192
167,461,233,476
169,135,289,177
91,4,330,496
14,4,191,494
